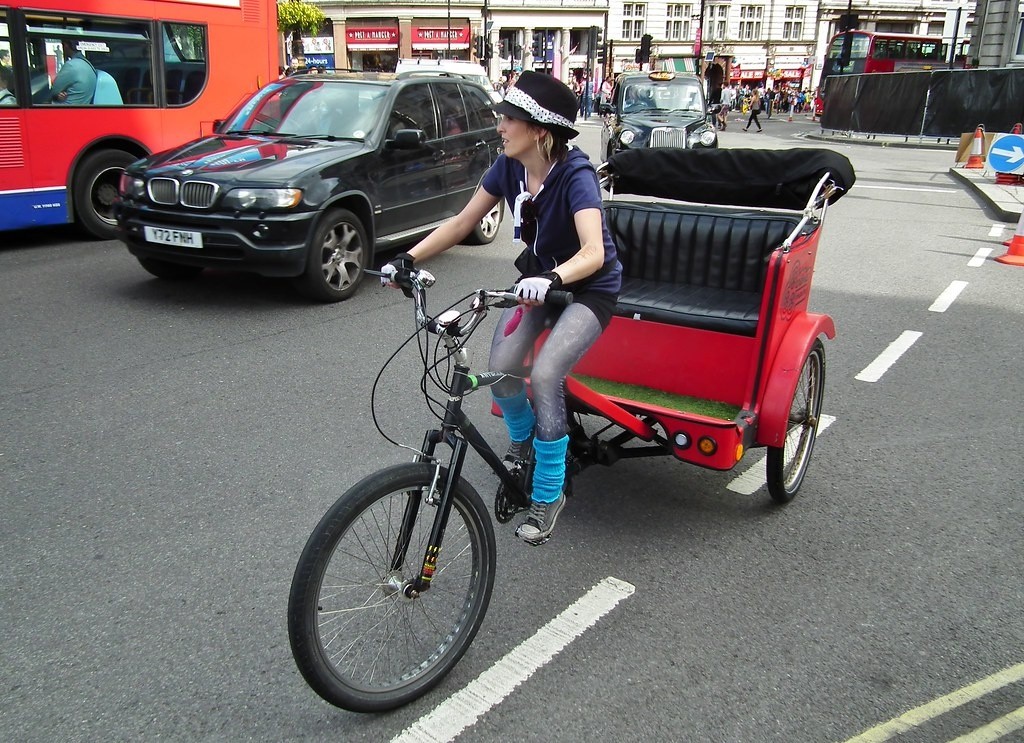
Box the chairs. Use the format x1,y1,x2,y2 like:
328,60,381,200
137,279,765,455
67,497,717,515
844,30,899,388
92,66,206,105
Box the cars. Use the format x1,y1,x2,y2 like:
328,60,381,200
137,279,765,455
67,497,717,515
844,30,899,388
600,70,722,162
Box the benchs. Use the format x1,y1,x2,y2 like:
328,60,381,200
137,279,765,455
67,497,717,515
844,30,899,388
601,200,818,338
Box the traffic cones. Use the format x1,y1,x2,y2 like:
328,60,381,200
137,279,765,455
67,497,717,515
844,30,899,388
995,205,1024,266
964,127,984,168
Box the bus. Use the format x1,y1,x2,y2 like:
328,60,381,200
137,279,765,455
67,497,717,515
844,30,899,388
0,0,280,240
814,30,970,115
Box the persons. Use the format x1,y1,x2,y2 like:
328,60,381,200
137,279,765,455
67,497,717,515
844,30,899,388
0,50,10,69
727,84,818,119
493,70,518,95
48,40,98,104
566,75,612,118
279,57,326,80
717,82,731,131
380,70,623,540
743,90,762,132
0,65,17,104
52,45,63,72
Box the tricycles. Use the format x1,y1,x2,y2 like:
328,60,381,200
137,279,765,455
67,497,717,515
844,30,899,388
287,148,857,711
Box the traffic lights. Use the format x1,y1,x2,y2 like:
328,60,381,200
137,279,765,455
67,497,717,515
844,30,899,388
473,35,483,58
597,43,607,65
486,44,493,58
532,33,543,58
591,26,602,59
641,36,653,63
497,39,508,60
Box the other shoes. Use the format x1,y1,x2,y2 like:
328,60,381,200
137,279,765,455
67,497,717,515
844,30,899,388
718,125,721,129
757,129,762,132
742,127,748,132
719,123,727,131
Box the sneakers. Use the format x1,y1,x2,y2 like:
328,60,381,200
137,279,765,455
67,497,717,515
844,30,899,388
502,427,535,474
515,490,567,543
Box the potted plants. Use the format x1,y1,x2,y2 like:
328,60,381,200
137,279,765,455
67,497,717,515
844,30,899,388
277,0,325,37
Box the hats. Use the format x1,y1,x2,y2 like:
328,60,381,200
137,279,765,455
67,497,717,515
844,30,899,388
487,70,580,141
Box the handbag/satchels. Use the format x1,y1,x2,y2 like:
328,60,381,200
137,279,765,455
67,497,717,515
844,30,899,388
756,108,761,115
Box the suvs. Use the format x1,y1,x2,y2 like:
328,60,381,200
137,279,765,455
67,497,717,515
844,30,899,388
113,58,504,301
